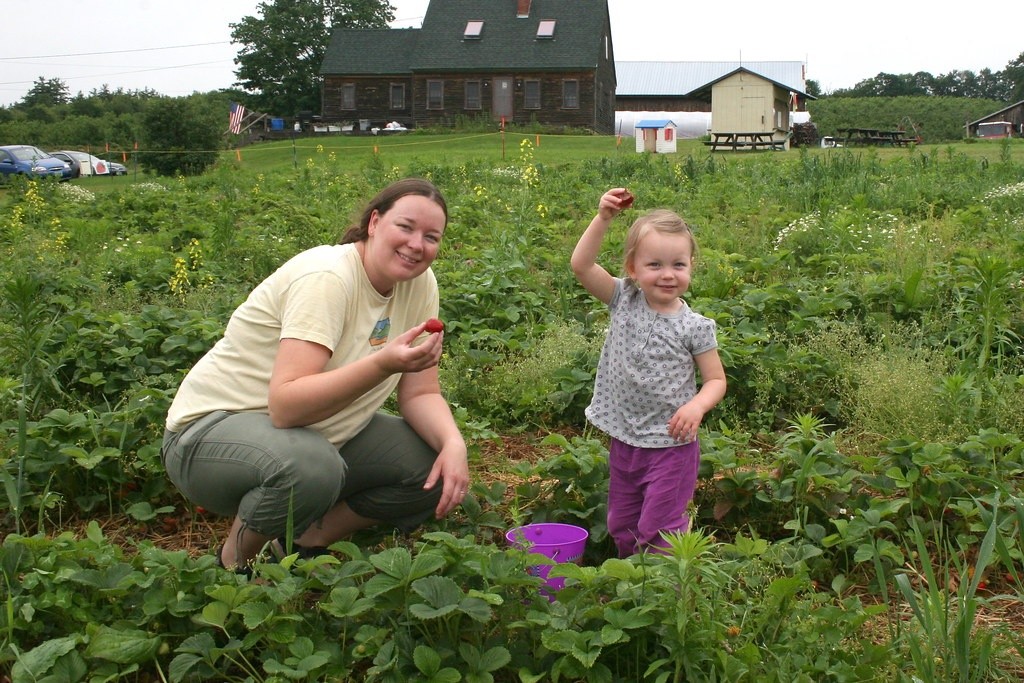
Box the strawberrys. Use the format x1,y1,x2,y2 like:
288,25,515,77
977,570,1022,589
615,189,634,204
117,480,207,533
424,318,443,332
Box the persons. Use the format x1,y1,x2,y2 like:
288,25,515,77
913,122,926,144
157,179,469,587
569,188,726,604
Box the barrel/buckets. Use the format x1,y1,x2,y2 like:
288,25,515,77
505,524,589,605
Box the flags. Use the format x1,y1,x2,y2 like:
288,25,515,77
229,103,244,134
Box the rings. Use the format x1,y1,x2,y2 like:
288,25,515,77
461,491,466,494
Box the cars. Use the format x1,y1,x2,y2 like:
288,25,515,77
48,150,127,179
0,145,71,182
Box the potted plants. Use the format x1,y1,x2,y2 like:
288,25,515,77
312,120,356,132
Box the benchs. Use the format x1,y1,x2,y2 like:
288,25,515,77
701,141,784,146
825,137,919,143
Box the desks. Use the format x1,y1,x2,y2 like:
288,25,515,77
708,132,777,152
832,128,908,148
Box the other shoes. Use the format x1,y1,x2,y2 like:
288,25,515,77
214,543,279,589
268,535,346,593
